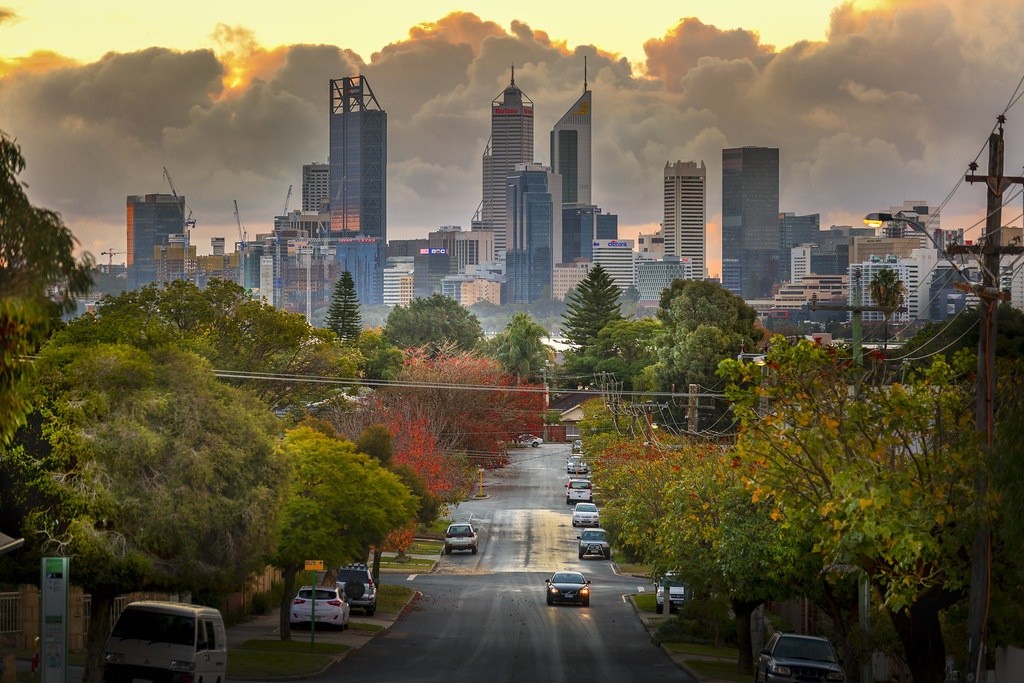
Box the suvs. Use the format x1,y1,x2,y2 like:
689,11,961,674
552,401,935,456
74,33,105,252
290,583,352,632
334,562,381,617
444,522,480,556
655,570,686,611
564,477,594,505
755,630,847,683
576,528,611,560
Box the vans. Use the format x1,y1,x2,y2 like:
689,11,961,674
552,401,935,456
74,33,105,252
100,598,228,683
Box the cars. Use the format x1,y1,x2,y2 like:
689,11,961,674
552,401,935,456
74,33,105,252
570,503,602,528
544,569,593,608
512,433,544,448
566,439,589,474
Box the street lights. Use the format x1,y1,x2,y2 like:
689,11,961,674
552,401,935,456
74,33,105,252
863,213,1004,683
584,385,608,411
648,422,700,619
